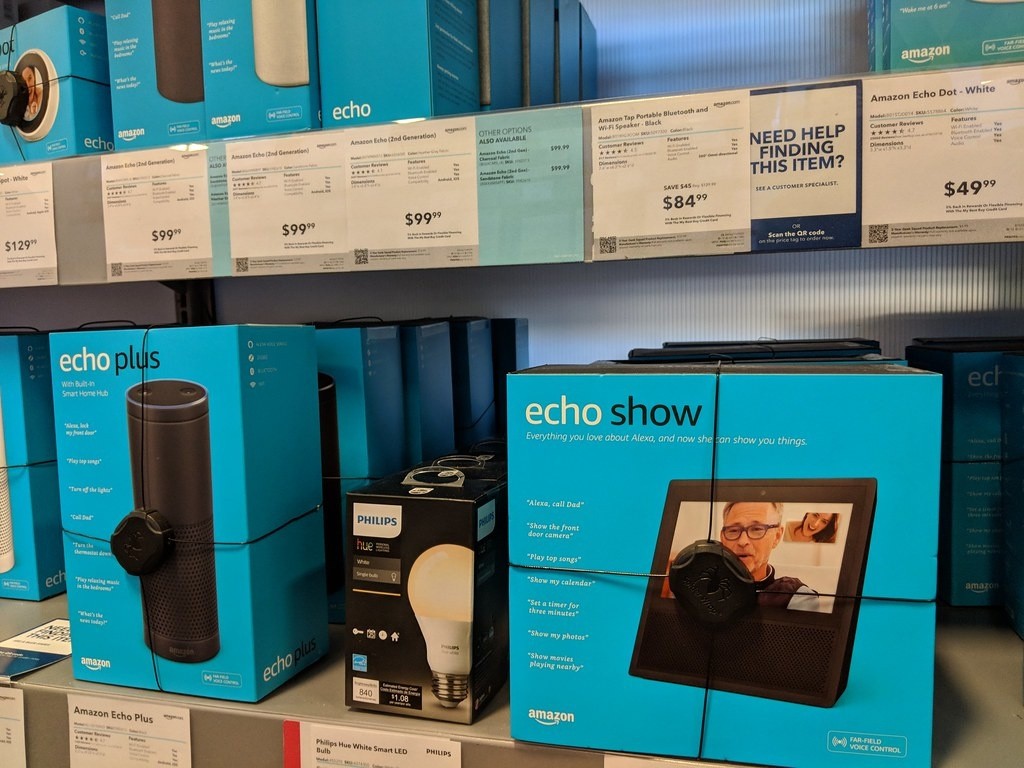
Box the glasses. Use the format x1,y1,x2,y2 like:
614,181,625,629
723,524,779,541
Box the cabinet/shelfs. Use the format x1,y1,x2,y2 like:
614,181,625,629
0,0,1024,768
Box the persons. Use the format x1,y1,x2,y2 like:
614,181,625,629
783,512,840,543
721,502,820,611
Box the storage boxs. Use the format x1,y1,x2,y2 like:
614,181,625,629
0,0,1024,768
